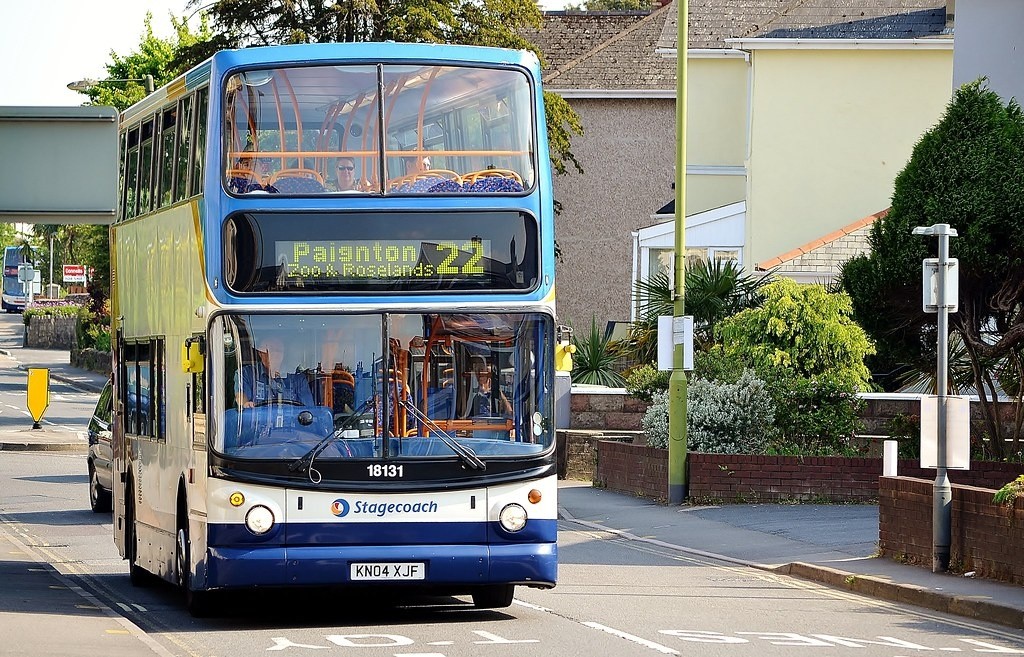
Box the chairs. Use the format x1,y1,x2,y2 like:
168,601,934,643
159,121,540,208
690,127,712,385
321,379,355,413
226,169,265,193
359,169,523,193
269,168,324,193
368,378,415,434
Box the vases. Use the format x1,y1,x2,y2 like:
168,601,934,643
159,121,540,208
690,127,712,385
998,378,1024,397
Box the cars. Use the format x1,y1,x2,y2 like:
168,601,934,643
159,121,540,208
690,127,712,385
86,379,114,513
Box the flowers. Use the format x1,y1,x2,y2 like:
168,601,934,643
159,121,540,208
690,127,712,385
993,364,1019,384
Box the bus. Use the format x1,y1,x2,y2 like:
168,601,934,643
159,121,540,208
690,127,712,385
116,44,558,620
2,246,45,313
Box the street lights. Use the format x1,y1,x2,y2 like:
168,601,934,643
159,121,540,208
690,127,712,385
912,224,970,574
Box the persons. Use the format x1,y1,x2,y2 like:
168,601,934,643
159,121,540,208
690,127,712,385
464,364,512,416
241,157,262,178
233,337,314,408
333,157,355,191
404,147,431,175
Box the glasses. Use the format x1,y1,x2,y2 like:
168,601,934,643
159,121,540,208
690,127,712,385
338,166,354,171
